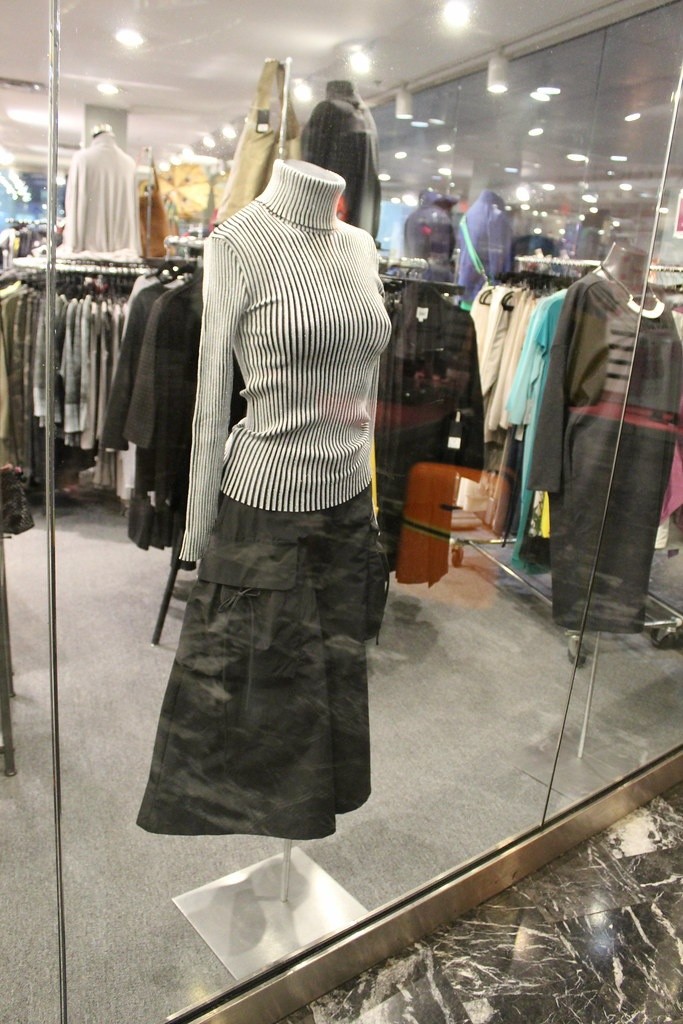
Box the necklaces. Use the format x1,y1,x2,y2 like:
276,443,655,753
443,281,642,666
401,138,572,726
601,261,665,319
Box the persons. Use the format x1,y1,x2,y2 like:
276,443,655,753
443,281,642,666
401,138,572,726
58,81,599,292
136,157,391,840
523,239,683,630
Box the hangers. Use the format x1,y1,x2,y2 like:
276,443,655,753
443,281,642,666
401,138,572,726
145,234,183,280
161,235,196,285
385,257,442,333
21,257,150,306
478,254,683,312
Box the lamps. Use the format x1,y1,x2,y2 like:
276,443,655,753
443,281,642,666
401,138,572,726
486,55,510,93
395,88,414,120
